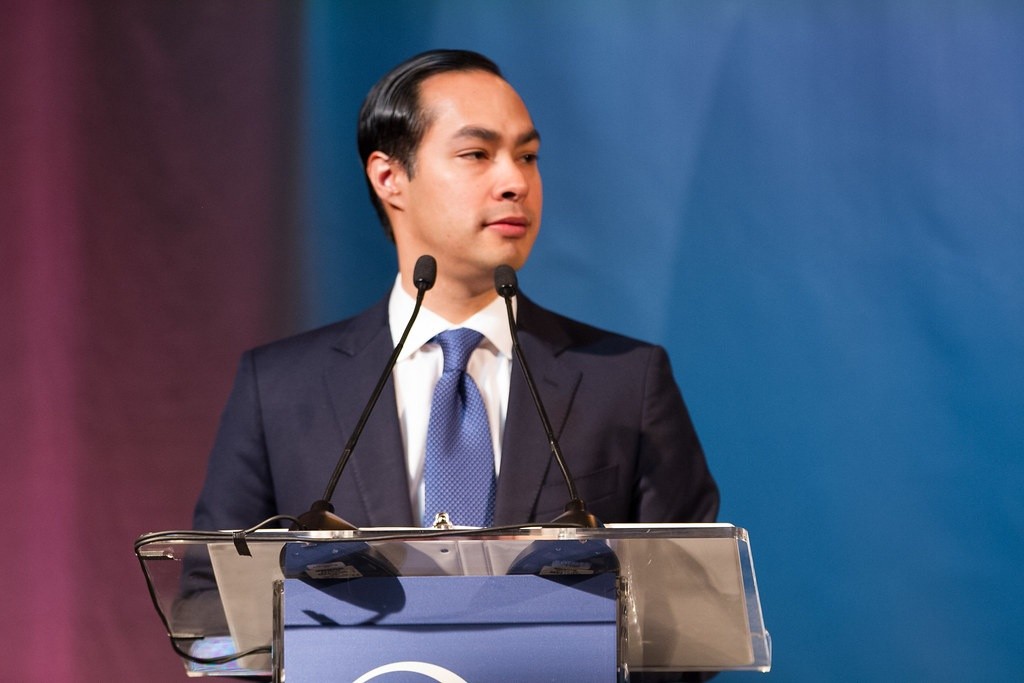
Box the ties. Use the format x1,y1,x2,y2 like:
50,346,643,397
423,327,501,528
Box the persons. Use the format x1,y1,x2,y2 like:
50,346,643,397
173,50,720,633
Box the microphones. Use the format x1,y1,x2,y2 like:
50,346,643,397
493,264,619,573
278,254,437,579
